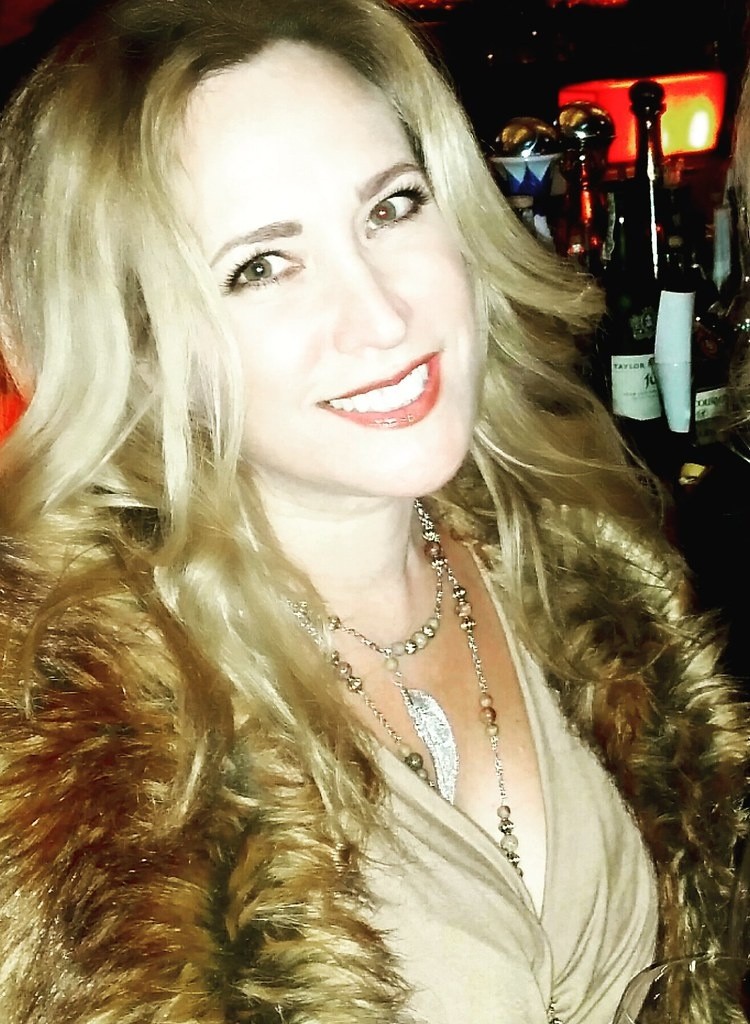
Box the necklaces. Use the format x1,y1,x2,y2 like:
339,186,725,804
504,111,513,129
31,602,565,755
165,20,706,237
284,498,523,876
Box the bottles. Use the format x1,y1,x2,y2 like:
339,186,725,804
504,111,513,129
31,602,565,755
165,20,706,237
507,195,750,486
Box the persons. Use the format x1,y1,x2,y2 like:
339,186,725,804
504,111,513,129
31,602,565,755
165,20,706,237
0,0,750,1024
